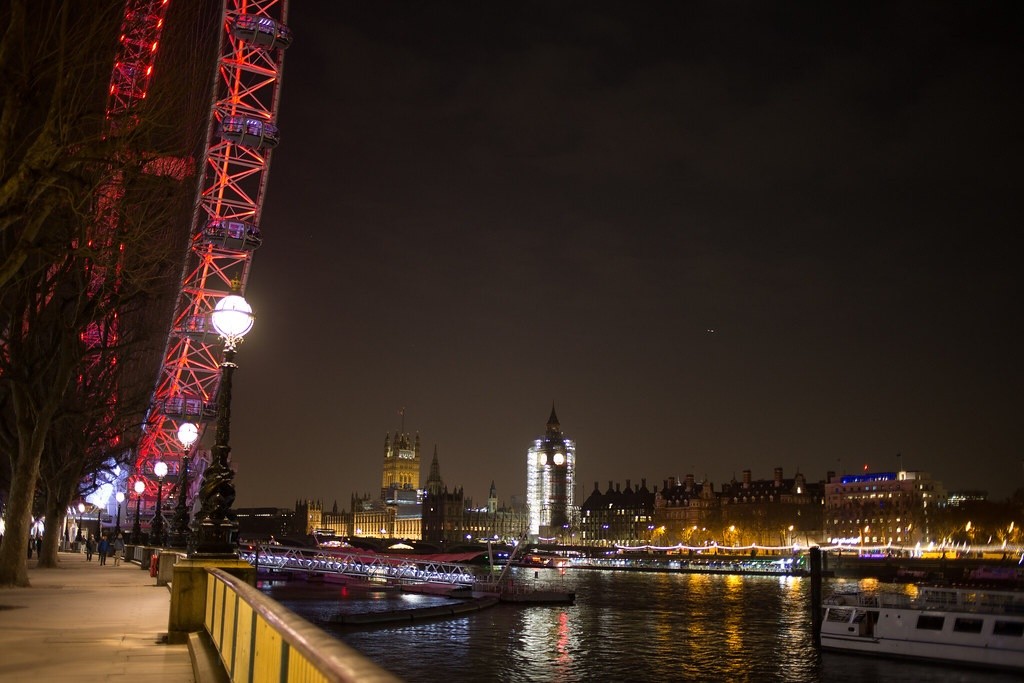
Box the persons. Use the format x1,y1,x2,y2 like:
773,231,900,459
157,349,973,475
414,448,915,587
98,536,112,565
27,535,43,559
114,533,124,566
86,535,96,562
81,535,86,554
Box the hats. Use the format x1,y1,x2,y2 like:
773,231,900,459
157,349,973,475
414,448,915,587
118,534,123,539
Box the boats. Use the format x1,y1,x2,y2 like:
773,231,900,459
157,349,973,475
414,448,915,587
820,583,1024,671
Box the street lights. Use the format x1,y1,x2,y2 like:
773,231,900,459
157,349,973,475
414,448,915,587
113,492,125,544
129,480,145,544
95,503,104,541
165,421,198,549
75,505,84,541
147,460,169,547
187,270,258,560
